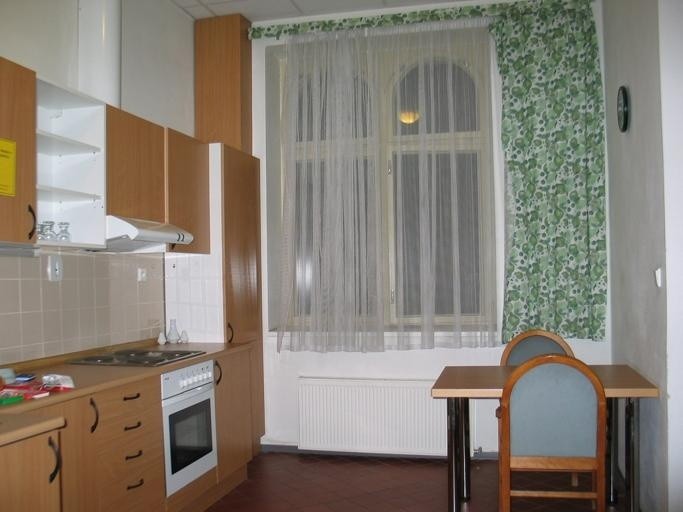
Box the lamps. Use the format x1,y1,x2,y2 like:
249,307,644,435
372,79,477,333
399,112,420,124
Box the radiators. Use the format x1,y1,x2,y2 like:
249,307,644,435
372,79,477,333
295,375,482,458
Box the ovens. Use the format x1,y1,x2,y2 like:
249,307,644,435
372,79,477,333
159,359,218,499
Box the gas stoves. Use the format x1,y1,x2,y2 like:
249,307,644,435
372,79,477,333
65,348,207,368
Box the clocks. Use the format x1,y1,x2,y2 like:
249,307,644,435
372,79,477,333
617,85,628,132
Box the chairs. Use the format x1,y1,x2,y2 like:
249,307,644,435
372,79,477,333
496,353,609,512
499,328,580,487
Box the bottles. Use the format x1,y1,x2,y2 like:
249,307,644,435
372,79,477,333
167,320,179,344
177,338,182,344
180,329,189,344
157,332,167,345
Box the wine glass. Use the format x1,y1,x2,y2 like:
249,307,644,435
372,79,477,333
36,220,71,242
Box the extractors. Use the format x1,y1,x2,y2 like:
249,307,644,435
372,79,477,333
83,214,194,255
